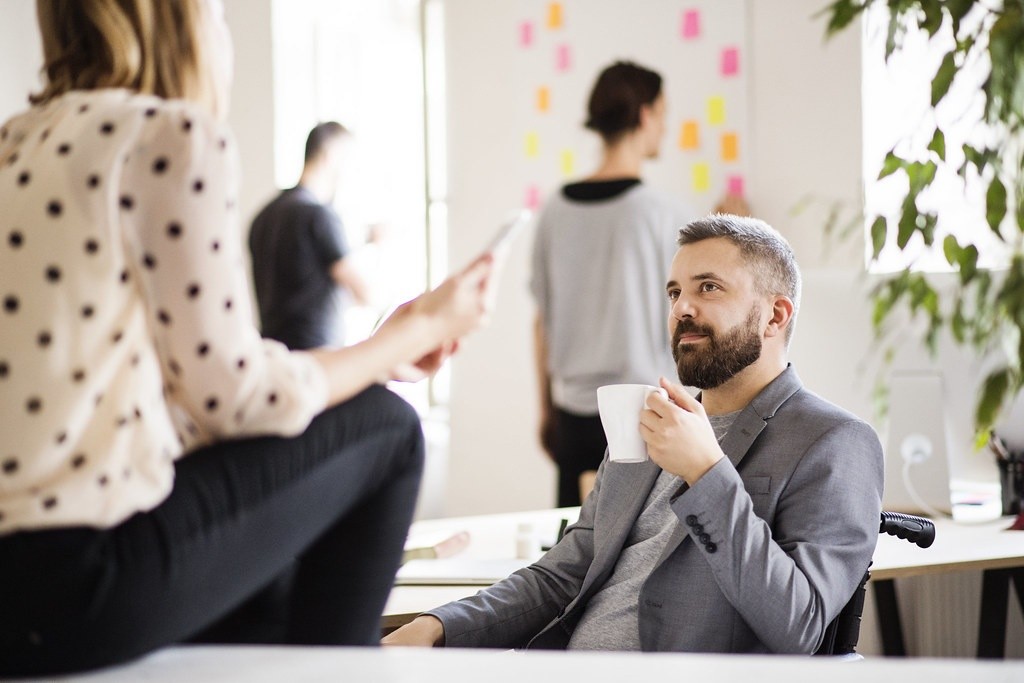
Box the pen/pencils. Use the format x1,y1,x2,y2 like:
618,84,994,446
989,429,1011,459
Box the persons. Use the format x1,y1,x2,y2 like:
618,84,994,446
377,212,885,655
535,74,703,509
0,0,491,683
243,119,371,351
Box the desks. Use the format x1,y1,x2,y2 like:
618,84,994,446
0,515,1024,683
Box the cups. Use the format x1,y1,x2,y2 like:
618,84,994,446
595,383,668,465
998,458,1024,515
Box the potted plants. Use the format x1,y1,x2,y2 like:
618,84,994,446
820,0,1024,516
